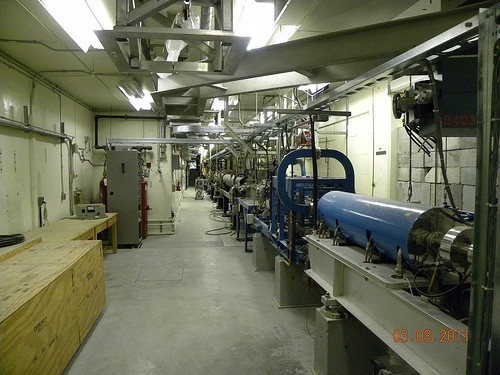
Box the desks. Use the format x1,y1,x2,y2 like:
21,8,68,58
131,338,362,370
0,212,118,375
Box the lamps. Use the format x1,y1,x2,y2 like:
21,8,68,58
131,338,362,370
117,77,144,99
